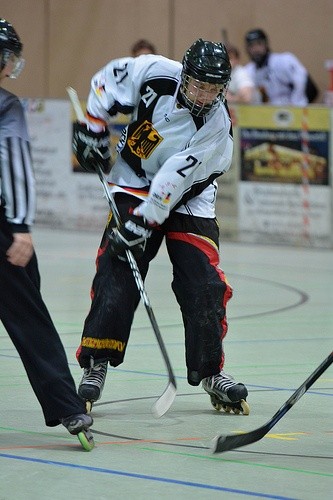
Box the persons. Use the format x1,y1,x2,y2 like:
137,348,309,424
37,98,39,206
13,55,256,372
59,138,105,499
0,15,98,449
227,27,323,105
73,37,250,415
131,38,156,57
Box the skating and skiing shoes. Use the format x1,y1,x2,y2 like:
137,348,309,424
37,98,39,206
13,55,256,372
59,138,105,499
202,372,249,415
78,361,108,415
59,413,95,451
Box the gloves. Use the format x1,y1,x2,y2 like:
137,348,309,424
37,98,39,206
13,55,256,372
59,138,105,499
108,214,152,263
71,121,110,174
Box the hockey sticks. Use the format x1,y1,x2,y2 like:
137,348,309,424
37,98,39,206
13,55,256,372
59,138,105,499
210,352,333,456
65,85,178,419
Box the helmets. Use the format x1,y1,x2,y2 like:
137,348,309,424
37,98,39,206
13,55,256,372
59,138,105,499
0,17,22,72
178,38,232,117
245,30,269,67
